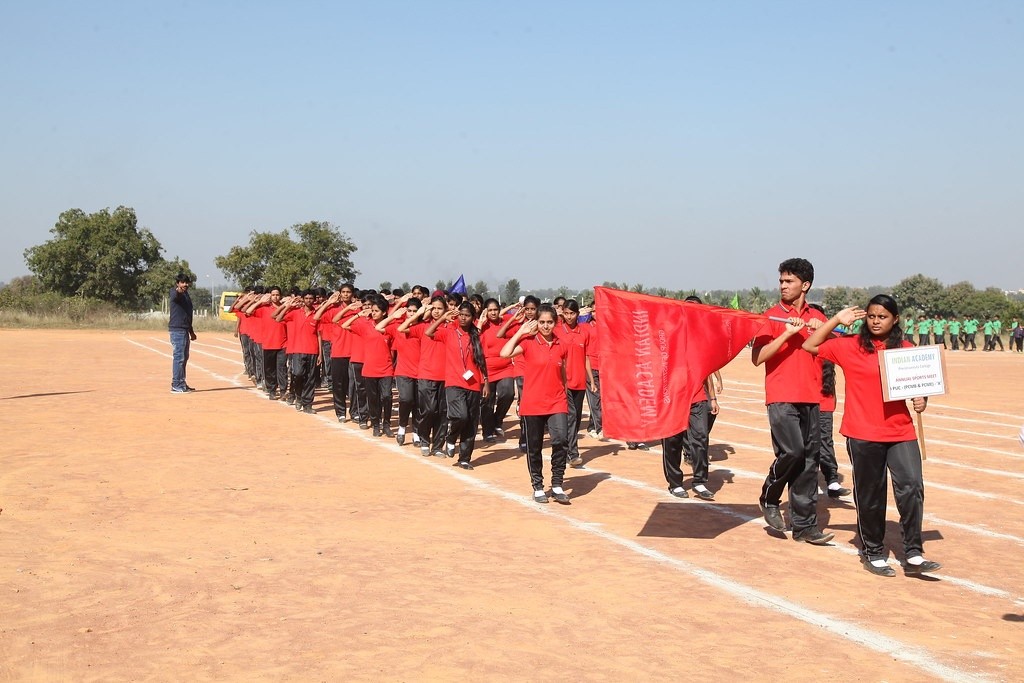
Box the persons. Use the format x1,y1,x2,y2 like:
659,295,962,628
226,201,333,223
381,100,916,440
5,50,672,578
809,304,852,498
168,274,196,394
838,314,1024,353
752,258,835,544
229,284,723,504
801,294,941,577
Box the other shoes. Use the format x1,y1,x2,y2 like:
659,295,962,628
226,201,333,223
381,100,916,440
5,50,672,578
692,485,713,498
828,488,852,500
496,427,506,438
247,373,368,431
759,503,785,531
551,486,569,503
519,443,526,452
570,457,582,468
458,462,473,470
904,557,941,576
446,441,455,457
373,431,382,437
484,434,496,443
186,384,195,391
412,432,420,447
864,561,896,576
794,530,835,543
396,425,406,444
382,427,394,437
636,442,648,450
171,386,190,393
421,447,430,456
671,487,689,498
533,490,548,503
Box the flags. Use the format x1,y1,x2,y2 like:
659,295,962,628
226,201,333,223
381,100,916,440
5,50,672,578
448,275,466,295
594,285,768,442
730,293,738,309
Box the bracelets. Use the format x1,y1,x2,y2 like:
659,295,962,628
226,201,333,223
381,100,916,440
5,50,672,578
357,314,359,317
711,398,717,400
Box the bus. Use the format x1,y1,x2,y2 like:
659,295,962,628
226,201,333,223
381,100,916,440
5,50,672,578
218,291,245,322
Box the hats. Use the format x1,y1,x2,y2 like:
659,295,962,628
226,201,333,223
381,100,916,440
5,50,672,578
431,290,445,298
